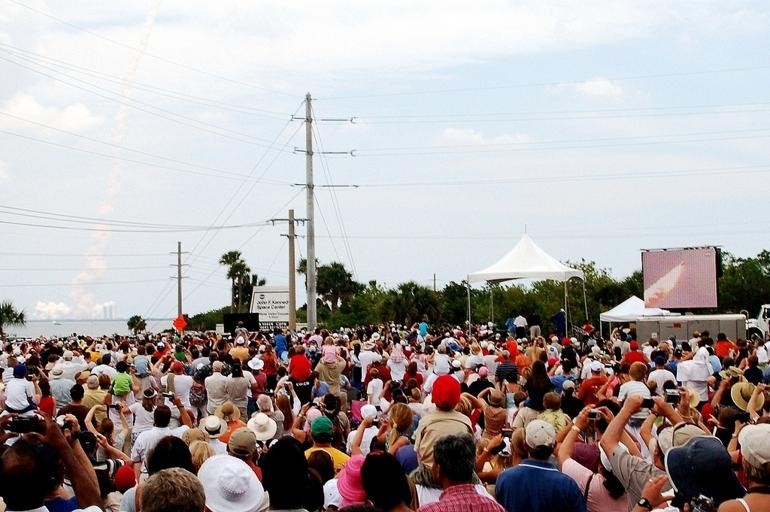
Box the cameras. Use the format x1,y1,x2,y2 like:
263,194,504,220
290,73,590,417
110,401,121,410
664,389,682,404
587,409,601,421
310,401,326,408
501,428,513,437
162,391,173,402
11,414,39,433
282,382,292,387
372,419,379,425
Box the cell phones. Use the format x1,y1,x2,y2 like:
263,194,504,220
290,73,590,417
640,398,655,408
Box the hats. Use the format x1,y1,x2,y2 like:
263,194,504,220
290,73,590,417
362,332,380,349
719,365,765,413
688,388,701,408
361,404,377,419
310,416,333,436
657,422,770,508
432,375,461,404
52,369,99,388
487,389,505,406
562,363,602,390
197,401,277,512
248,357,264,370
525,420,555,449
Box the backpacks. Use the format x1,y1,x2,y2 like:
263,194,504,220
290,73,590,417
324,410,341,449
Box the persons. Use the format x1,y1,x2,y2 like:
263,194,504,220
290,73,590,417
1,308,769,512
